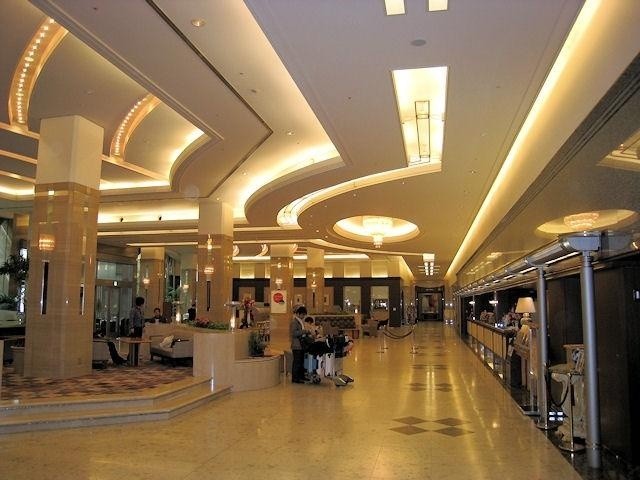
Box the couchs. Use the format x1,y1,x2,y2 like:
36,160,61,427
150,332,192,368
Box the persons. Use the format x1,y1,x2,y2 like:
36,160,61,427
303,318,315,329
188,303,197,320
290,306,307,385
151,308,168,323
569,348,574,363
573,350,579,366
505,315,515,327
126,297,148,368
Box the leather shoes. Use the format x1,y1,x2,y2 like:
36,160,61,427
292,375,311,384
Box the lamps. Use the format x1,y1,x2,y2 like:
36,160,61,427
514,297,536,327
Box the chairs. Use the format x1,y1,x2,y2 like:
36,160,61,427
107,341,128,366
